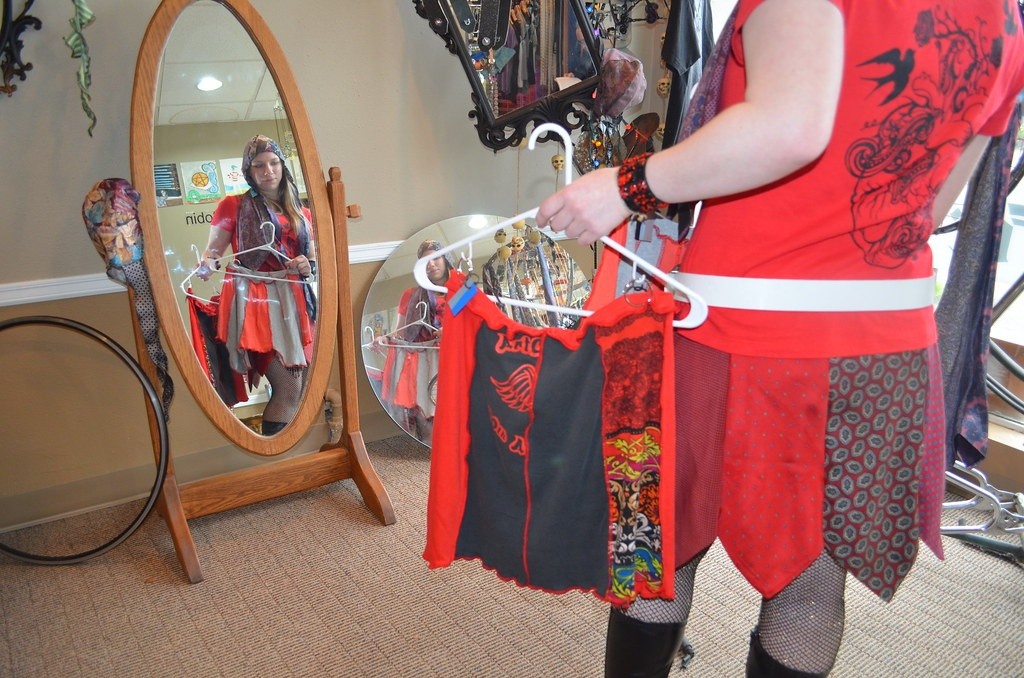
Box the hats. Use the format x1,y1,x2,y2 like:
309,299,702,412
83,178,141,277
242,134,294,193
417,239,456,269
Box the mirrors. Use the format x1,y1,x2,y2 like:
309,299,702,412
131,0,340,457
416,0,606,151
357,217,592,451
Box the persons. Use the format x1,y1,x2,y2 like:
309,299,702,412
536,0,1024,678
193,134,316,434
372,239,457,438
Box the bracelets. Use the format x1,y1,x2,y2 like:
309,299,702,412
619,155,666,214
208,257,221,270
386,336,391,340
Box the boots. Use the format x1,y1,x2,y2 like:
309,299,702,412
745,625,829,678
604,606,687,678
262,419,287,436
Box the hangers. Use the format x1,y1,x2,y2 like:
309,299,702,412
180,243,222,304
209,223,313,285
412,120,709,331
362,322,389,373
375,300,441,353
